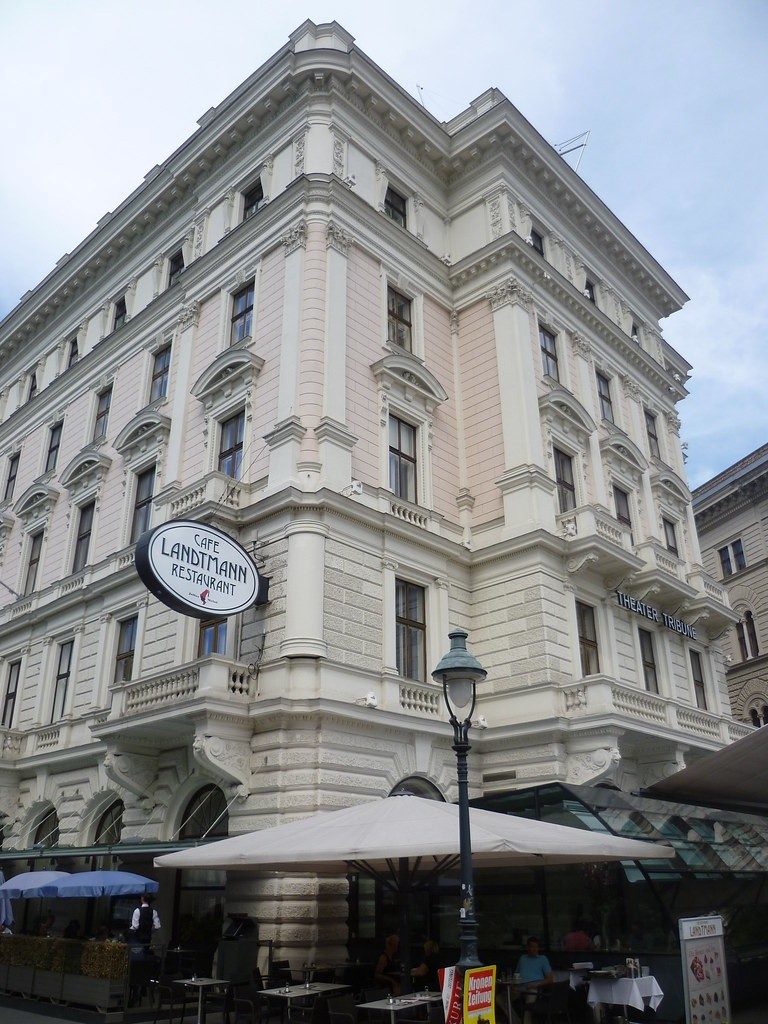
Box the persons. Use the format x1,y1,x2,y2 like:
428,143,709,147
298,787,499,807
564,925,589,947
496,937,553,1024
627,921,653,951
0,924,11,933
39,924,52,936
129,894,161,997
373,935,448,995
65,920,135,942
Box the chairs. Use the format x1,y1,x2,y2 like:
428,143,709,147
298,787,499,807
153,975,199,1024
234,980,269,1024
427,1007,445,1024
520,980,572,1024
286,992,342,1024
201,974,248,1016
308,968,338,984
250,968,279,989
329,993,359,1024
364,990,400,1024
269,960,294,984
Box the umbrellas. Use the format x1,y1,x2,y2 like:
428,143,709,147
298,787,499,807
39,870,159,921
630,723,768,816
0,871,71,916
0,869,15,926
155,791,676,933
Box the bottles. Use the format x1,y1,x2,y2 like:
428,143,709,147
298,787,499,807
387,994,393,1004
305,979,309,989
285,983,289,992
192,973,196,982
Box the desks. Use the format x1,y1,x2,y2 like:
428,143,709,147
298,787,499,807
353,990,442,1024
256,982,352,1024
279,965,337,984
585,974,664,1023
497,977,535,1024
171,977,232,1024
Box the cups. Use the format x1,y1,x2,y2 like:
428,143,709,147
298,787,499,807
513,972,520,979
641,966,649,977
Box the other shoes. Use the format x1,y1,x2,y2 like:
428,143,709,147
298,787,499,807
140,988,148,998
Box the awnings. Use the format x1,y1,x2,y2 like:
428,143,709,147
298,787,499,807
0,836,226,859
